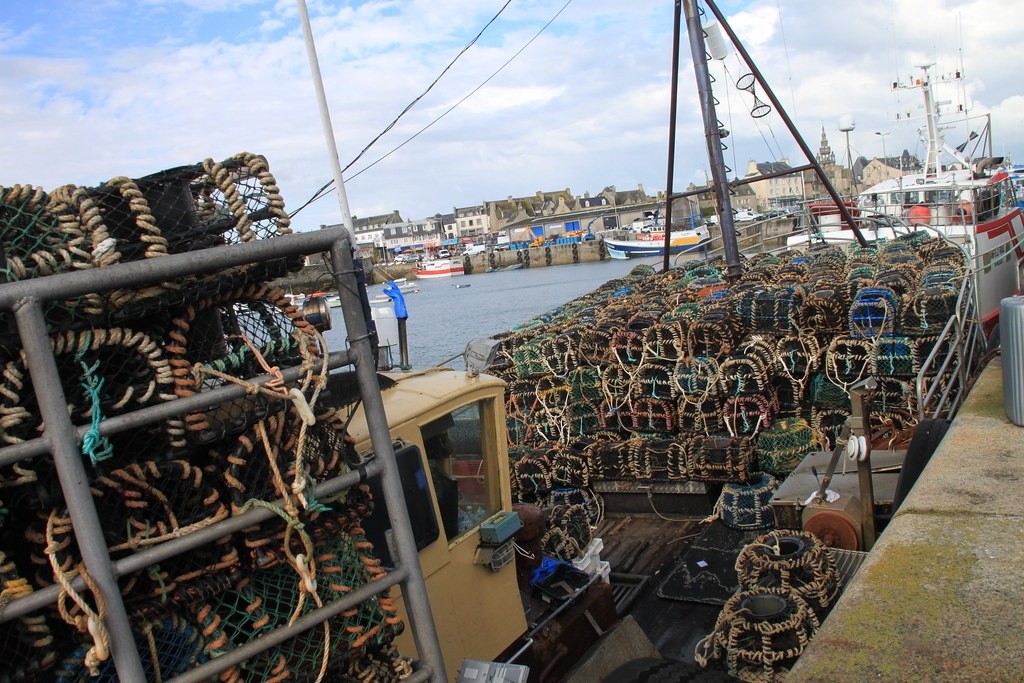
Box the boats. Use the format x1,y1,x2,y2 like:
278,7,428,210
785,46,1024,346
411,250,465,279
602,193,710,260
387,278,419,296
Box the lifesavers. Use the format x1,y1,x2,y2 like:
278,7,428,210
544,247,552,266
572,243,578,263
599,241,605,258
464,256,470,274
524,249,530,267
490,253,496,269
517,251,523,263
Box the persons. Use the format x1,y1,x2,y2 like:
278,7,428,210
415,454,443,539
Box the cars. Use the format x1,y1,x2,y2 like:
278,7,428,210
735,206,784,222
393,240,485,263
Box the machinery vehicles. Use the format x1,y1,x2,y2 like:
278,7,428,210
515,227,545,247
